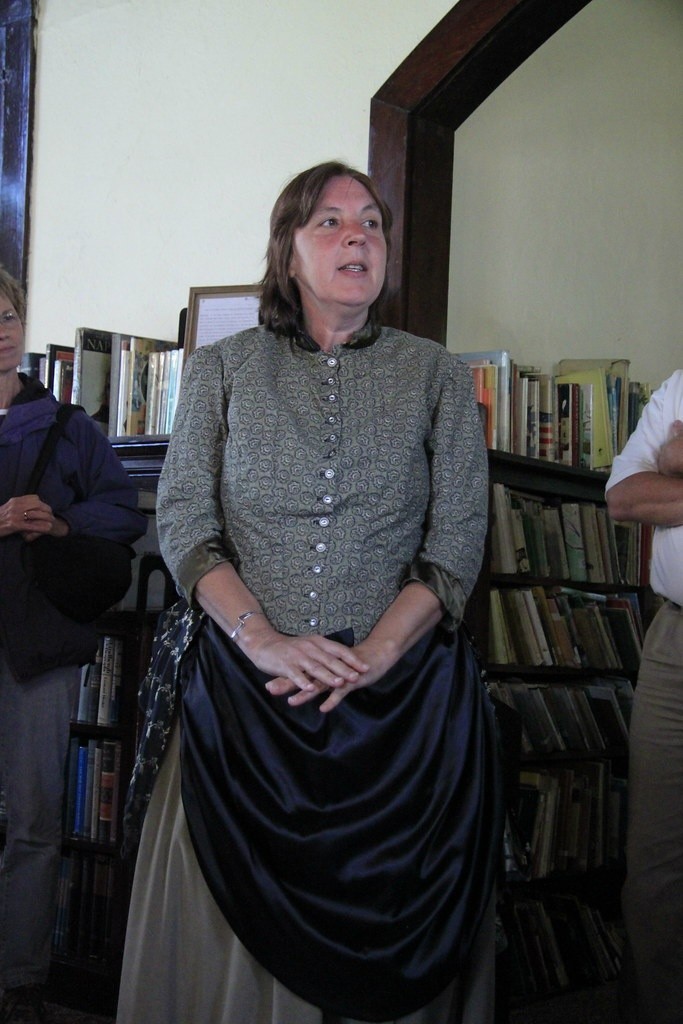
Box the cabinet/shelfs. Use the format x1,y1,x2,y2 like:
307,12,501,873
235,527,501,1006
46,430,655,1024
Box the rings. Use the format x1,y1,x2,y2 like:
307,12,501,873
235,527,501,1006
24,511,27,519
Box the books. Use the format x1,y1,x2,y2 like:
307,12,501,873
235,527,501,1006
65,737,123,842
453,353,665,994
69,635,123,724
54,854,114,962
20,327,183,438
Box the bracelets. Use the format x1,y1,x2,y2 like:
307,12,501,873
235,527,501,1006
230,611,264,642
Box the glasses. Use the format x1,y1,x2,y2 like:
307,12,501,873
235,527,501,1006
0,309,23,328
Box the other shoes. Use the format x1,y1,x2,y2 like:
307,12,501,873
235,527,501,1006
1,985,43,1024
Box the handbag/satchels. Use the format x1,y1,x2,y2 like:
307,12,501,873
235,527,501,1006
21,403,136,620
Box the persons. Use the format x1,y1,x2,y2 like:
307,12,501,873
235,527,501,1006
0,264,149,1024
605,368,683,1024
116,162,503,1024
